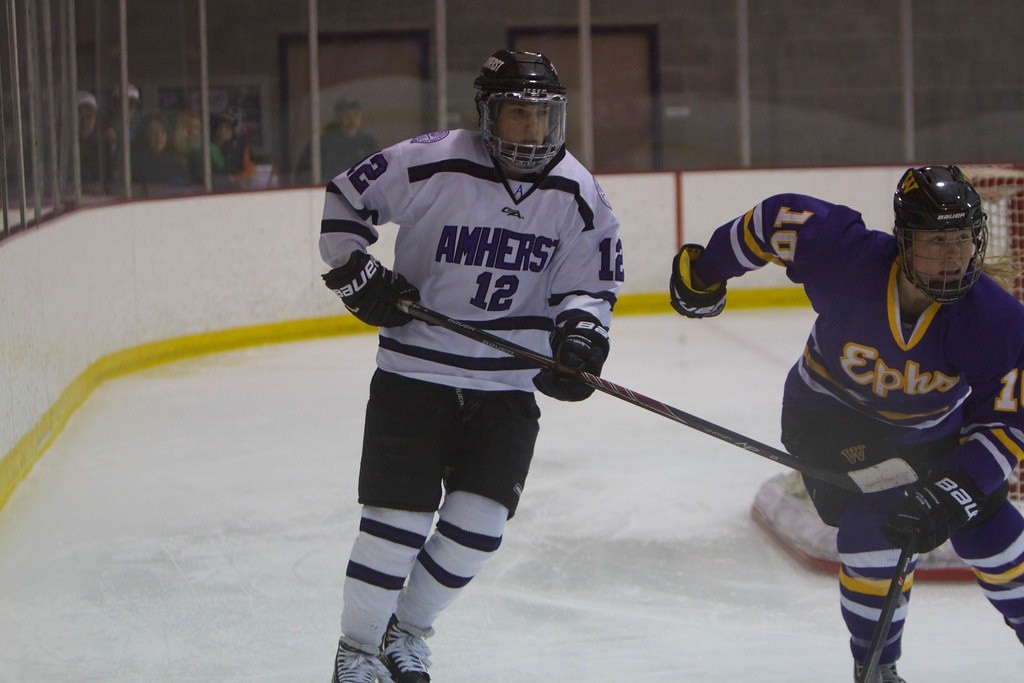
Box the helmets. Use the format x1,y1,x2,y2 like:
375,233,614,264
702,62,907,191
893,165,988,303
474,48,567,173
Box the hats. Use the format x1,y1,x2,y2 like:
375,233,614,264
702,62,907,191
111,83,139,99
335,96,363,111
77,90,97,107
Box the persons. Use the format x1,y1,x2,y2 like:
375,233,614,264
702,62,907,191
669,165,1024,683
317,48,629,683
292,96,380,186
72,81,254,199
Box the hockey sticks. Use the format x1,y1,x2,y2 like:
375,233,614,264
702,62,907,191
395,298,920,495
857,466,935,683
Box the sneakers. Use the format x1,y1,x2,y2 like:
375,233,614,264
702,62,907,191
332,635,381,683
854,658,905,683
378,612,435,683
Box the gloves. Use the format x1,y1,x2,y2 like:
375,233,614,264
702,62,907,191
670,244,727,318
880,473,987,553
532,319,610,402
321,249,421,327
